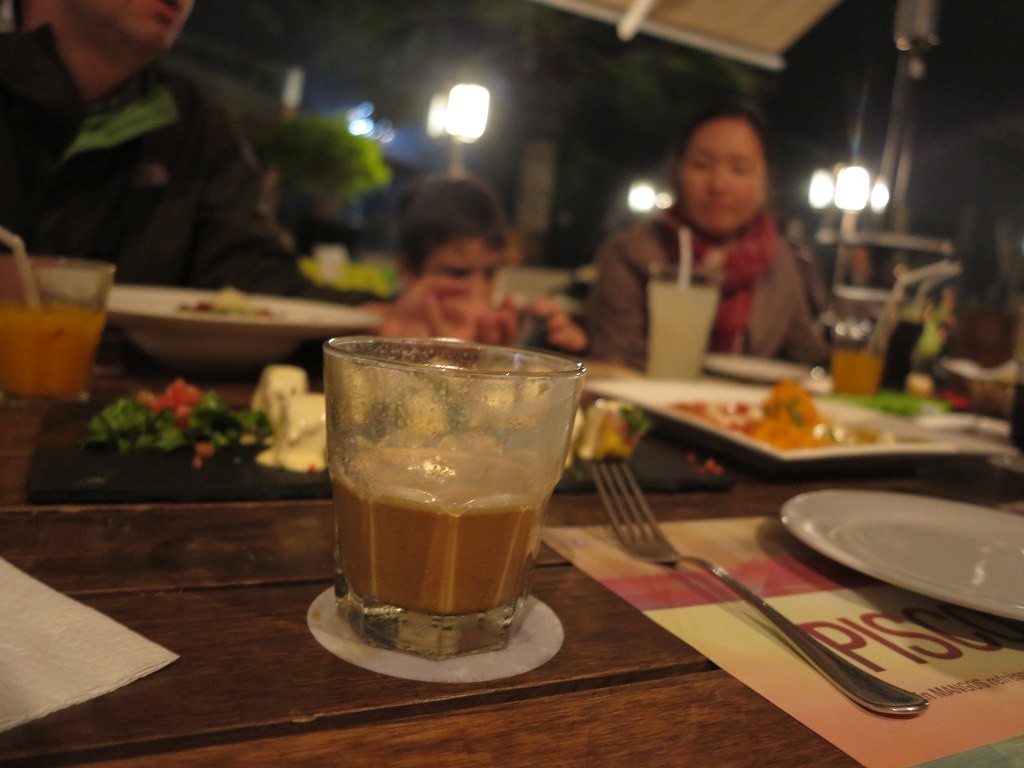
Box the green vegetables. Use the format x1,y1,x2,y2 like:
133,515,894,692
83,390,274,450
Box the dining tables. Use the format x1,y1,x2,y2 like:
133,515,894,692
0,331,1024,768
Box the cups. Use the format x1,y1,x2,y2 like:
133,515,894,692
881,296,933,392
643,277,721,380
318,333,585,662
0,253,117,402
831,285,904,394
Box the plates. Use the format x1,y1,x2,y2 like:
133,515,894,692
700,351,817,387
105,283,386,382
779,481,1024,634
581,376,1010,471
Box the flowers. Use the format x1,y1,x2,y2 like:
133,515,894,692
93,382,265,468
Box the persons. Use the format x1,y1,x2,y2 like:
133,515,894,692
586,98,834,378
0,0,520,373
398,176,586,359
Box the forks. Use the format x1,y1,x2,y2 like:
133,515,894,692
588,455,931,718
